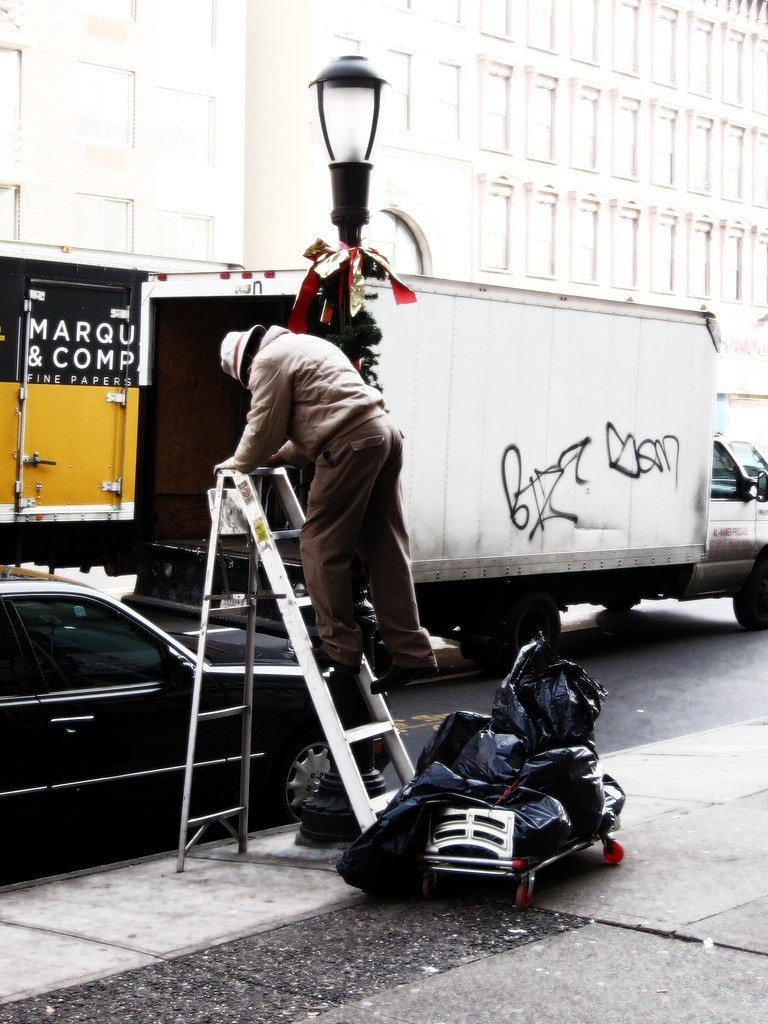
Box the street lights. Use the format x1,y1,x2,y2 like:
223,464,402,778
307,54,394,668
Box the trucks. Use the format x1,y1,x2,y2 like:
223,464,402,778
0,239,249,527
126,258,768,668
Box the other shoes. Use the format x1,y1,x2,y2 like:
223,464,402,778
293,648,361,675
370,665,439,695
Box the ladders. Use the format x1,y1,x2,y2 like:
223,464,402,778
175,461,415,871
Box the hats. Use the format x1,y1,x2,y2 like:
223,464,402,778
220,325,267,388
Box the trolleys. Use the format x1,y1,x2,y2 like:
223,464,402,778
413,806,626,912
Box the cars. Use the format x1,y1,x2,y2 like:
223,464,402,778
0,566,391,827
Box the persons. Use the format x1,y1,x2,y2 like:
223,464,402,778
211,325,439,695
